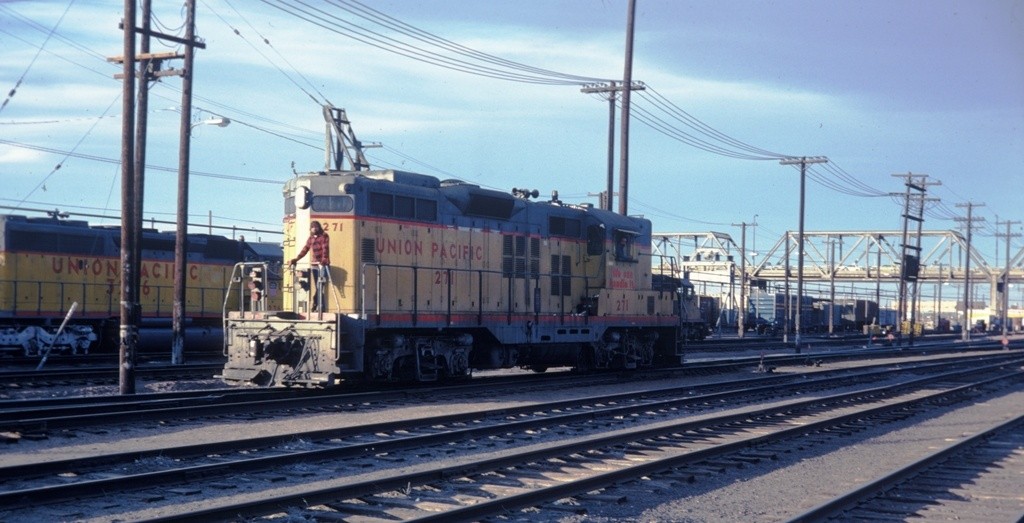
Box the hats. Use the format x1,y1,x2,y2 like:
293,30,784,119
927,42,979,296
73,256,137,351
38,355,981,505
295,266,306,271
253,268,261,272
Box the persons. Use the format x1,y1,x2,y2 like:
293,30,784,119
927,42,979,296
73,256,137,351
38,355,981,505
287,220,330,312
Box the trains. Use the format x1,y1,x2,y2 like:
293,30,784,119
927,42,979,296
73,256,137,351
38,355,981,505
0,209,283,330
210,104,691,393
673,264,714,342
